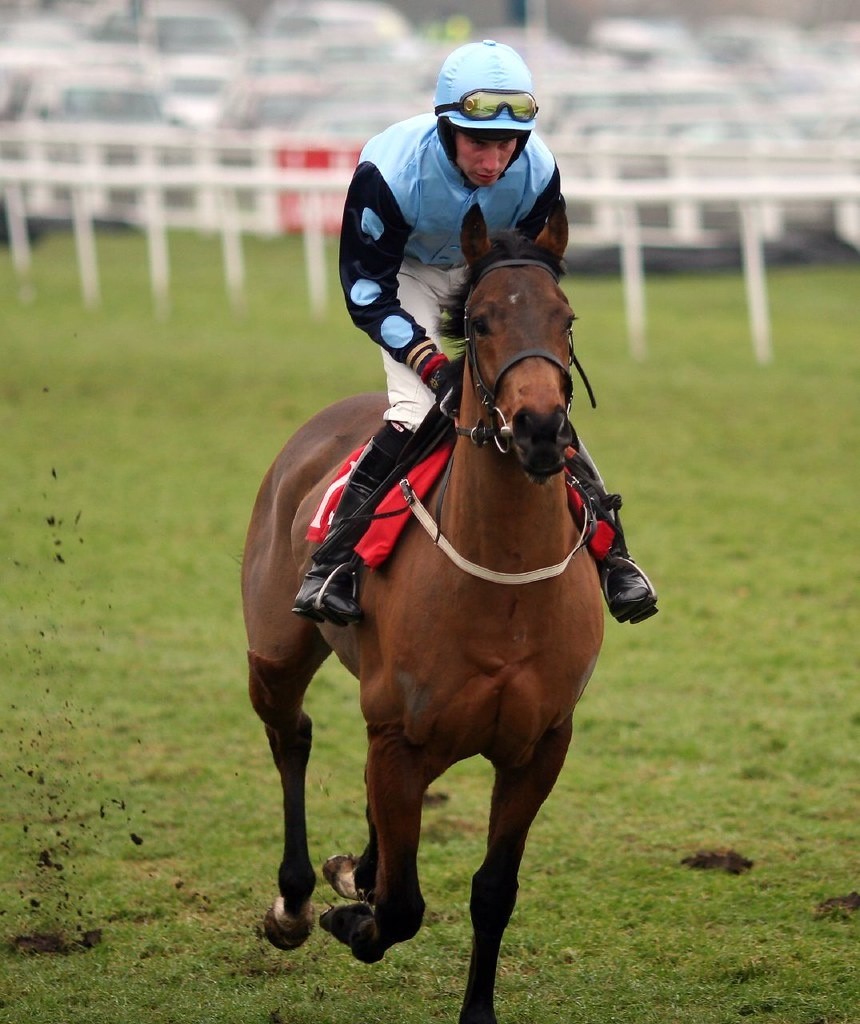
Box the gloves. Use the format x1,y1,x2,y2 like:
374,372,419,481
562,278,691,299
427,360,464,418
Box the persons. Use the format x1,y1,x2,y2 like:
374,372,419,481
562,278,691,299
291,42,668,630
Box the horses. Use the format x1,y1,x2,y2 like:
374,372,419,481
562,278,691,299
240,191,605,1024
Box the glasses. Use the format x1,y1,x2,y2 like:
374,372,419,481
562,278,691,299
435,89,538,123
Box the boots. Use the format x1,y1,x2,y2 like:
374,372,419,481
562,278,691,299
293,437,395,623
564,437,656,624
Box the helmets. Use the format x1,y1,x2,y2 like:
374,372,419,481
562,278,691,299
435,40,539,173
